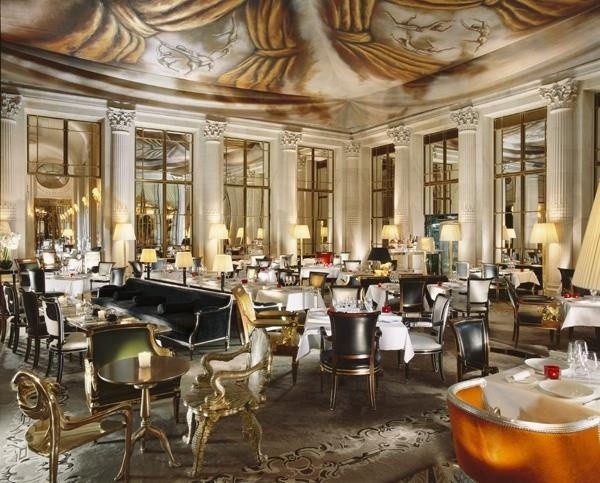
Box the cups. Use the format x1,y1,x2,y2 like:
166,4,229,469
57,295,118,321
139,367,151,380
544,364,561,379
138,352,151,367
447,270,459,286
437,280,442,286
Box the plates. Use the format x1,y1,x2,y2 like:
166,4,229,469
540,379,594,399
565,298,584,301
525,357,570,372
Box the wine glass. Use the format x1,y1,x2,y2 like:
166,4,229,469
282,275,296,288
590,289,600,299
331,296,373,311
567,338,597,384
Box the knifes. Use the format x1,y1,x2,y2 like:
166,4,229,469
582,397,600,404
